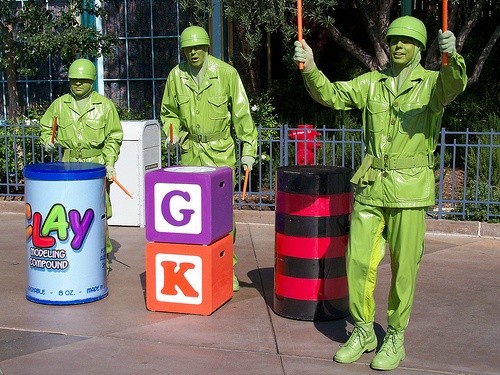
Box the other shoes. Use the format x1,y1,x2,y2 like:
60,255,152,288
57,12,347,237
106,253,109,276
232,273,240,291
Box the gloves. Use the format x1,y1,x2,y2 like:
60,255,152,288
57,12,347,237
242,154,256,173
164,136,180,152
293,38,316,73
105,166,116,183
45,136,60,153
438,28,457,59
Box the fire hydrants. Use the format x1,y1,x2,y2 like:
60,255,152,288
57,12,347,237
288,124,322,167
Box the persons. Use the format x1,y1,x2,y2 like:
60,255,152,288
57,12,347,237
160,26,258,292
293,16,467,370
38,58,124,280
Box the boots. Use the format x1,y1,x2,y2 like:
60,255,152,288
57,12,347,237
334,322,378,364
370,327,406,370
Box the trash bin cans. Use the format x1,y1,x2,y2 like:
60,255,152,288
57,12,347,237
108,120,161,228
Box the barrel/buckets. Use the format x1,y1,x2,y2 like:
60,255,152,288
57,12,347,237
272,163,355,319
21,160,107,306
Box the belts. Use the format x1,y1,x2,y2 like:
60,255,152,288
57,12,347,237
364,153,434,171
72,147,103,159
188,132,227,143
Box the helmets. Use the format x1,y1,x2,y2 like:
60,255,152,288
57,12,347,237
386,15,427,52
180,26,210,50
67,58,96,81
